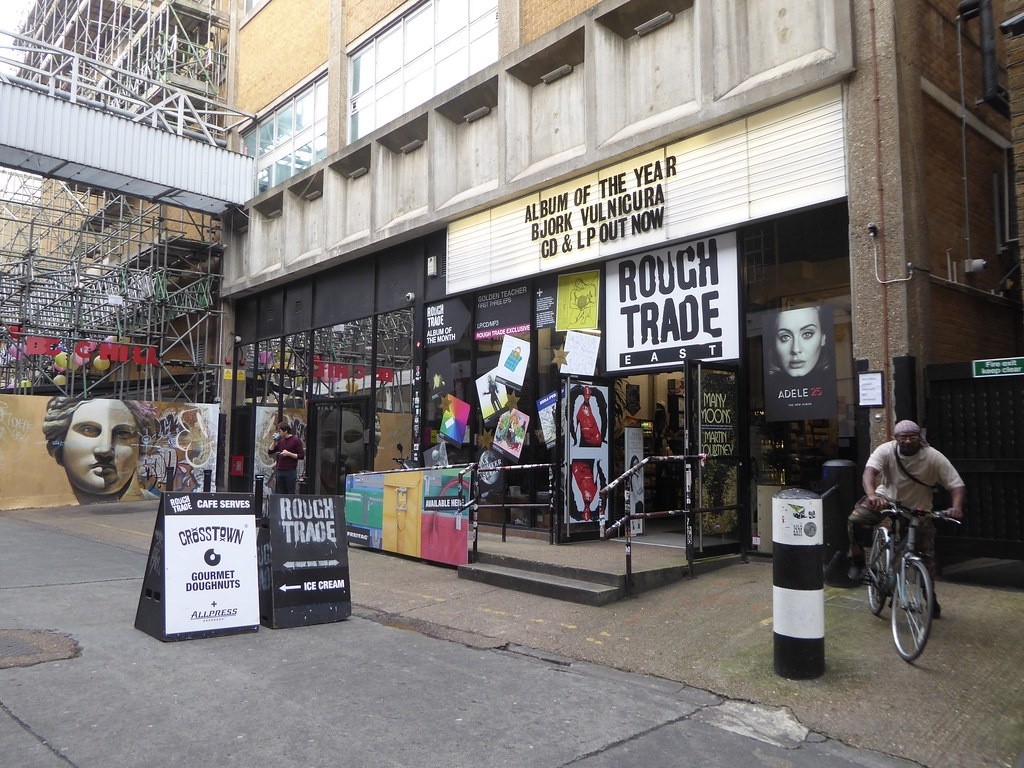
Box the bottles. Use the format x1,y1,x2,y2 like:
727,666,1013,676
769,465,774,482
781,469,786,484
760,439,784,450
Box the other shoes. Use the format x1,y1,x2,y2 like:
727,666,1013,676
848,564,866,579
922,590,940,618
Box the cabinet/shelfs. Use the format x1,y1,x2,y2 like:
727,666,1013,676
777,419,829,485
644,378,685,510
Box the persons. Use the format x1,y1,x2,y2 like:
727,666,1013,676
766,306,836,419
846,420,967,620
268,423,305,495
629,455,643,513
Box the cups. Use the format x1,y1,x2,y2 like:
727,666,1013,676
274,433,281,441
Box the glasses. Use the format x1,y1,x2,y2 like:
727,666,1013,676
897,436,919,442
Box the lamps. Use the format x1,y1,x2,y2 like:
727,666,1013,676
238,224,248,233
540,65,572,83
399,138,422,154
268,209,282,219
634,11,674,37
348,166,368,178
305,191,322,202
462,106,491,122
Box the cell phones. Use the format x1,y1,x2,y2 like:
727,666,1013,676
275,451,281,455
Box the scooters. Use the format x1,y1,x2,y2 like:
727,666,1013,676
358,442,419,475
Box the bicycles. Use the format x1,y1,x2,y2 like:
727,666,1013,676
862,502,962,662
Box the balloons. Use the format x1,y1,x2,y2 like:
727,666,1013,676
5,323,133,391
